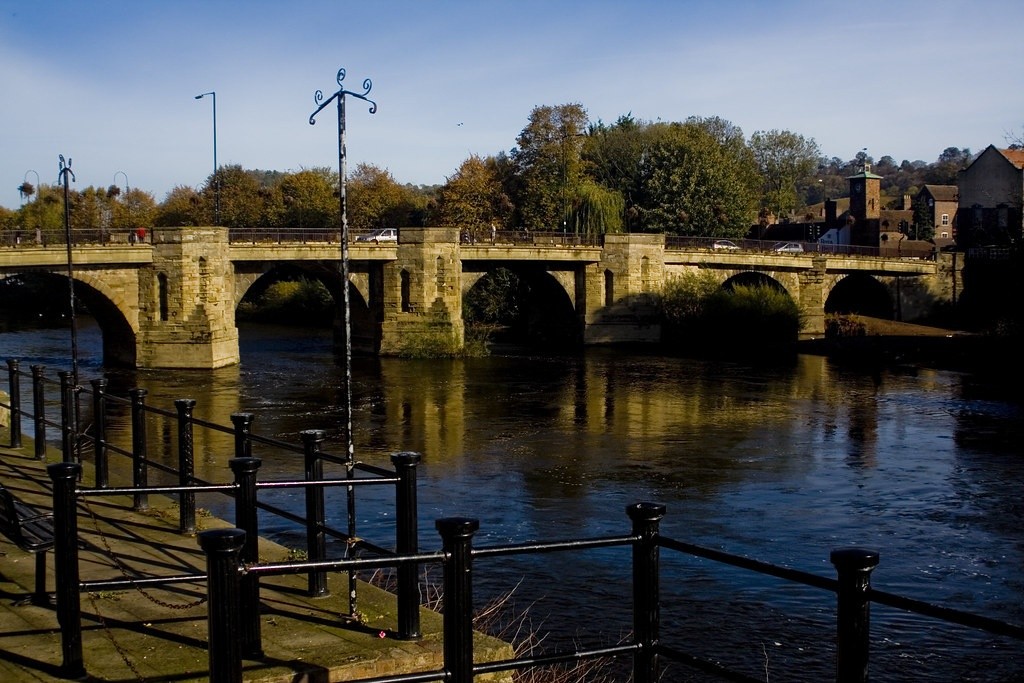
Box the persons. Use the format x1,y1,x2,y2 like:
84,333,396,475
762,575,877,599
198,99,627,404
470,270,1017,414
12,225,21,245
30,224,41,244
137,224,146,244
490,222,496,244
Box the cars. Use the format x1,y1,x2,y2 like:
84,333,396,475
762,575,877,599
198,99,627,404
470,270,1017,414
357,228,398,244
770,242,804,256
707,240,741,251
982,245,1003,259
897,222,903,233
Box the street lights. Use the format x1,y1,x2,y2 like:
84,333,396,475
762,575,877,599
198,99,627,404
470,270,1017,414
562,133,585,246
194,91,221,226
